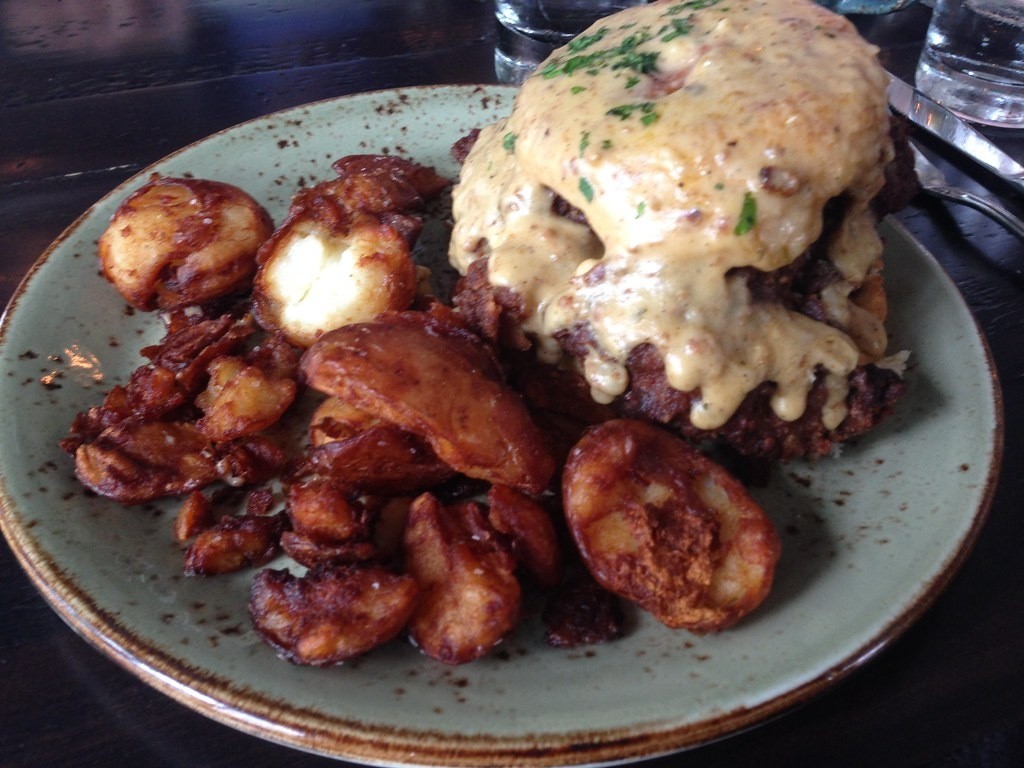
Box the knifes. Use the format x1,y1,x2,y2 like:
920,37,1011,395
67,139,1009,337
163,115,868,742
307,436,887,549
881,65,1024,193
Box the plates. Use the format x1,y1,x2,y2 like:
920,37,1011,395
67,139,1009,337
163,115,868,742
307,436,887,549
0,83,1005,768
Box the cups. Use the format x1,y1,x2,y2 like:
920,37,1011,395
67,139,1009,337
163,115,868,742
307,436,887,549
913,0,1023,129
494,0,647,87
